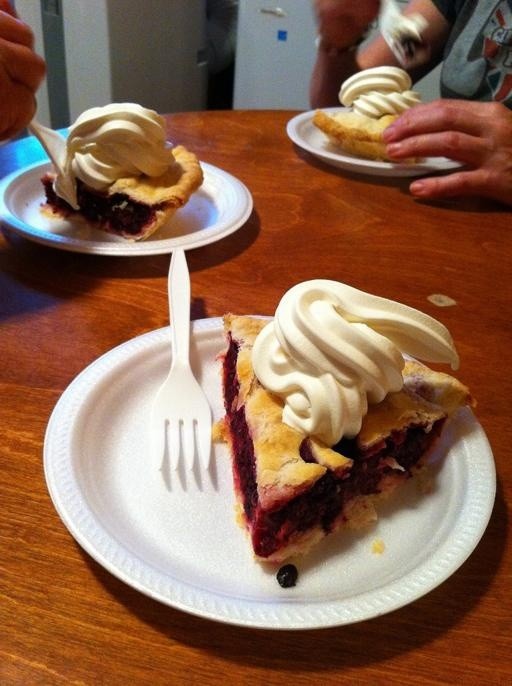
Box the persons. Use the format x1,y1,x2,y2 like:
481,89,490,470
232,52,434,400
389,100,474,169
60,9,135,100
306,0,511,201
0,0,47,143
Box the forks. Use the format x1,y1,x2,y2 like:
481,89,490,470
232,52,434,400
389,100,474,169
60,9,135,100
150,245,213,474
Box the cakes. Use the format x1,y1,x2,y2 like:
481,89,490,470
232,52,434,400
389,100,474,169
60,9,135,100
209,279,475,563
29,102,204,241
312,66,426,165
379,1,430,69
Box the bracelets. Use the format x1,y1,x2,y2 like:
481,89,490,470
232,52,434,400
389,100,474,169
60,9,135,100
317,26,372,58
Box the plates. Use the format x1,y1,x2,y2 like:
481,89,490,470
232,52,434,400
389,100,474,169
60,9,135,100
40,315,497,633
287,106,465,179
0,153,254,258
